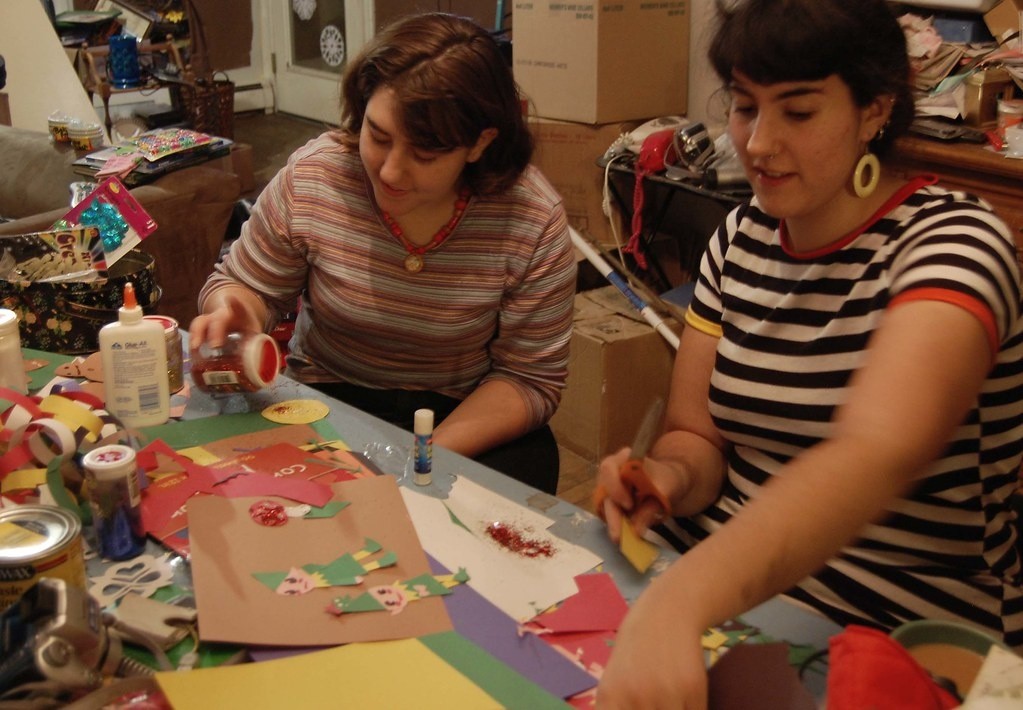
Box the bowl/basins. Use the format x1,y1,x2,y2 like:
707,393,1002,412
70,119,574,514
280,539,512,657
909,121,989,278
888,621,1015,705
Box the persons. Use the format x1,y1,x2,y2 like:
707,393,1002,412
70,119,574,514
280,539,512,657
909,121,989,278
186,10,579,497
588,0,1023,708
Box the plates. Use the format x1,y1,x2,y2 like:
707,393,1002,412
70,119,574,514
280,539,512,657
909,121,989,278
54,11,121,26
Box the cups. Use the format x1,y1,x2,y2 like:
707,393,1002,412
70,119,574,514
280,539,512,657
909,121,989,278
111,36,140,89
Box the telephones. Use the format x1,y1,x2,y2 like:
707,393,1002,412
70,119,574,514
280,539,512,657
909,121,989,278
623,113,694,169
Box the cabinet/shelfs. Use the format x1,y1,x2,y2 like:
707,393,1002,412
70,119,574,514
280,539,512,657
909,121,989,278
78,32,185,138
894,132,1023,273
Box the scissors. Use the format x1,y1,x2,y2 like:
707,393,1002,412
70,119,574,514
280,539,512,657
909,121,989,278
594,397,672,529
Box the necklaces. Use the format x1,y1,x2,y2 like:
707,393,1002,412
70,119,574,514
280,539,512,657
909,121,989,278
379,184,471,274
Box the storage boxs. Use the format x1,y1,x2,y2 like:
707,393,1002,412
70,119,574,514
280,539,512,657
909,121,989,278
509,0,691,295
899,0,1023,126
545,284,684,463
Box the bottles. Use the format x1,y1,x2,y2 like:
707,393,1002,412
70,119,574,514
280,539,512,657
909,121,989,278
98,283,170,426
83,445,148,559
144,315,185,395
191,327,279,397
0,309,28,396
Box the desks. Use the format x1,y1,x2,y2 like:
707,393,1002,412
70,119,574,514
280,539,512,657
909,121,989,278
0,324,849,710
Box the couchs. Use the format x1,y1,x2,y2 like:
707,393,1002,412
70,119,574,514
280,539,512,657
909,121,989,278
0,125,240,333
